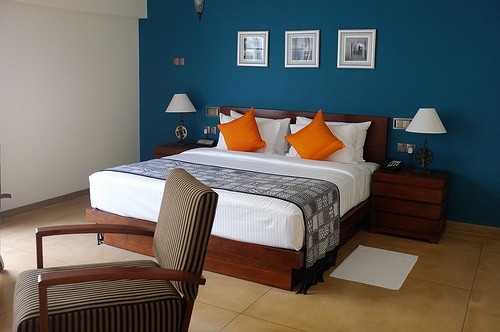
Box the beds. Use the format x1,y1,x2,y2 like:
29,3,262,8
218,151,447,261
85,107,388,295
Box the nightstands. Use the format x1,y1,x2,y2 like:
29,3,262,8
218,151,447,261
154,143,192,158
367,166,450,244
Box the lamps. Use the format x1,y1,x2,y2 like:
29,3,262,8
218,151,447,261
204,126,216,135
165,94,196,145
405,108,447,174
194,0,205,20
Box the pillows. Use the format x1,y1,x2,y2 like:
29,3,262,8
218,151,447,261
284,109,344,160
230,111,291,154
297,116,371,163
218,107,266,151
216,113,280,155
286,123,357,162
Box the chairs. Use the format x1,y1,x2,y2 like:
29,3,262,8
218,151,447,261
13,168,219,332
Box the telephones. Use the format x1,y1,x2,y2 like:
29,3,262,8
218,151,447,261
380,157,404,172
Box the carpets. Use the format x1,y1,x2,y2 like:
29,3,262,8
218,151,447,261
329,245,418,291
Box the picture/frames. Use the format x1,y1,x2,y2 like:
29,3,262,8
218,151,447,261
337,29,377,68
285,30,320,68
237,31,269,67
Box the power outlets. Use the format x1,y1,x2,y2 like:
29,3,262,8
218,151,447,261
398,143,415,152
